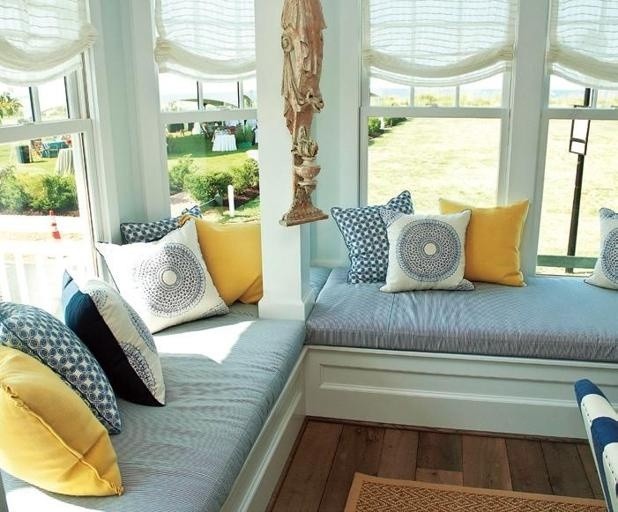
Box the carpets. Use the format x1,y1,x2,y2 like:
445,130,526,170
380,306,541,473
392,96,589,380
346,474,608,512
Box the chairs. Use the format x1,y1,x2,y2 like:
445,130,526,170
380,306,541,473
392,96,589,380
572,376,618,510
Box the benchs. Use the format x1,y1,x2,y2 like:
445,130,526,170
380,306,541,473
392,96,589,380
301,266,617,445
2,300,306,512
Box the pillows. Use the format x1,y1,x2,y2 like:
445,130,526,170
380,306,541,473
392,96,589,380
330,190,530,295
583,207,618,294
